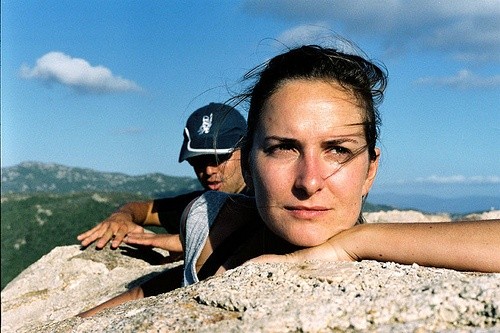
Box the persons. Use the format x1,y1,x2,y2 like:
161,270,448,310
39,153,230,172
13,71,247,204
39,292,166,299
70,27,500,318
76,102,247,253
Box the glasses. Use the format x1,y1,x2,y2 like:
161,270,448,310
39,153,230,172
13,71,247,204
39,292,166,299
186,149,237,167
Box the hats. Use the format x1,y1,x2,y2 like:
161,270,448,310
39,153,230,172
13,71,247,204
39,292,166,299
178,102,248,163
